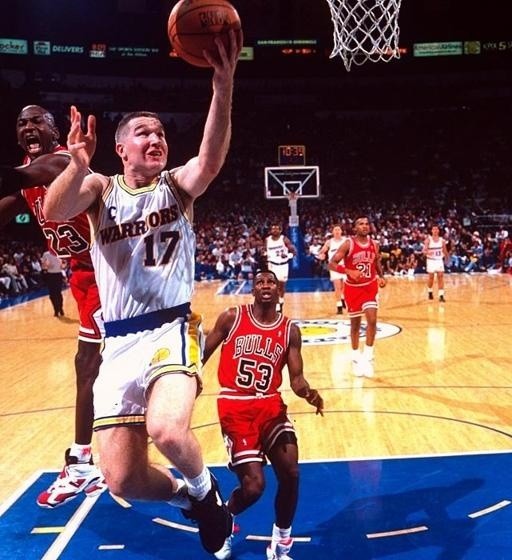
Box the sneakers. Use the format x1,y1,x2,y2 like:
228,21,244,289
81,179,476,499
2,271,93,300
182,473,235,560
277,303,282,313
439,295,445,302
338,298,346,314
353,356,374,378
37,447,108,509
266,540,295,560
429,292,433,299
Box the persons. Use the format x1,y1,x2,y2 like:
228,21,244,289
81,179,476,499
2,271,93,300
325,214,387,379
202,267,324,559
1,70,509,316
43,27,243,556
0,103,107,510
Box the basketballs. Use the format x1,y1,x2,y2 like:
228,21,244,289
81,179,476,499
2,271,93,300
165,1,242,69
